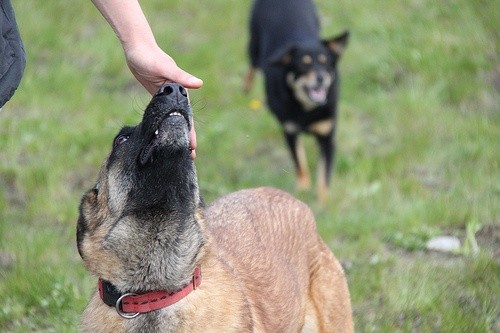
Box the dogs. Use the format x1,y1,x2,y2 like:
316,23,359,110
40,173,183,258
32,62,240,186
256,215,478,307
76,79,355,333
241,0,350,206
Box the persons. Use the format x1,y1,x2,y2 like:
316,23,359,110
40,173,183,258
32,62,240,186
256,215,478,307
0,0,206,161
